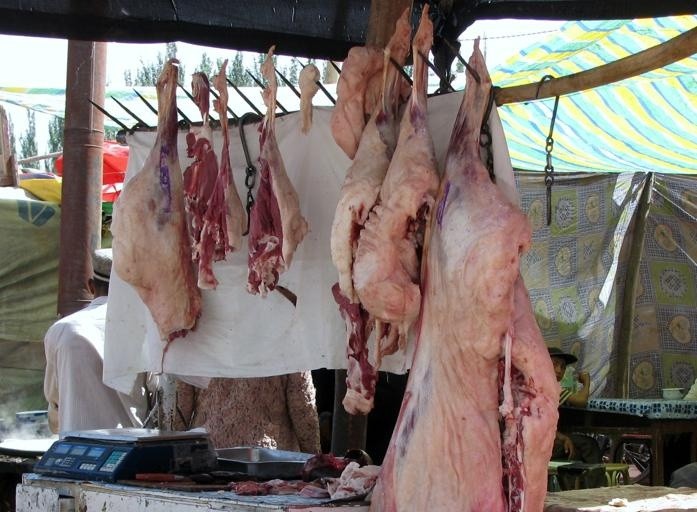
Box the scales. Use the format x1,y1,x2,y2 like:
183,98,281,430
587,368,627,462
32,426,219,483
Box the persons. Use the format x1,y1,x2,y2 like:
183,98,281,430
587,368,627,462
546,345,609,489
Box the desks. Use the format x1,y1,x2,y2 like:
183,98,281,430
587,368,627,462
15,473,331,512
559,398,697,485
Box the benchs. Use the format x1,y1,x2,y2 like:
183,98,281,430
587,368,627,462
548,461,612,489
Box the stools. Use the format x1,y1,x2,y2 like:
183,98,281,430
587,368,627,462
605,462,629,487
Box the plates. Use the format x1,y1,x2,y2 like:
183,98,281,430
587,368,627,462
213,447,316,478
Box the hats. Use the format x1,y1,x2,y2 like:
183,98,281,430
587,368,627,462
91,247,113,282
547,346,578,364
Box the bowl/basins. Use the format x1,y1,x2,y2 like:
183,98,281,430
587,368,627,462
661,389,685,400
